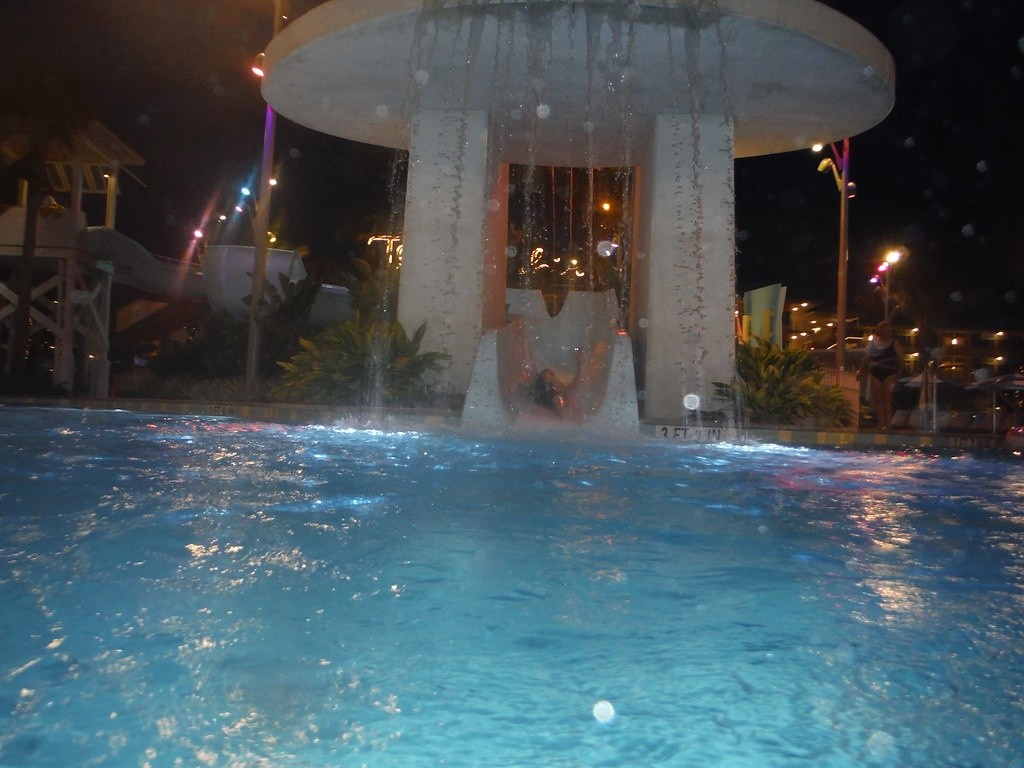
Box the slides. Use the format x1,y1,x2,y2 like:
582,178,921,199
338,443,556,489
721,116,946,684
81,224,641,438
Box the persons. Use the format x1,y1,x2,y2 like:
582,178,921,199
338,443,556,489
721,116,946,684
854,320,905,432
528,368,565,414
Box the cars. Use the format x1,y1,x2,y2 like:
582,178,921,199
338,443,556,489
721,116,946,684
897,362,1024,414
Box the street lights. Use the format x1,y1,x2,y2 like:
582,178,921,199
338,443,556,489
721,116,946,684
812,138,849,386
870,248,899,330
244,0,288,400
